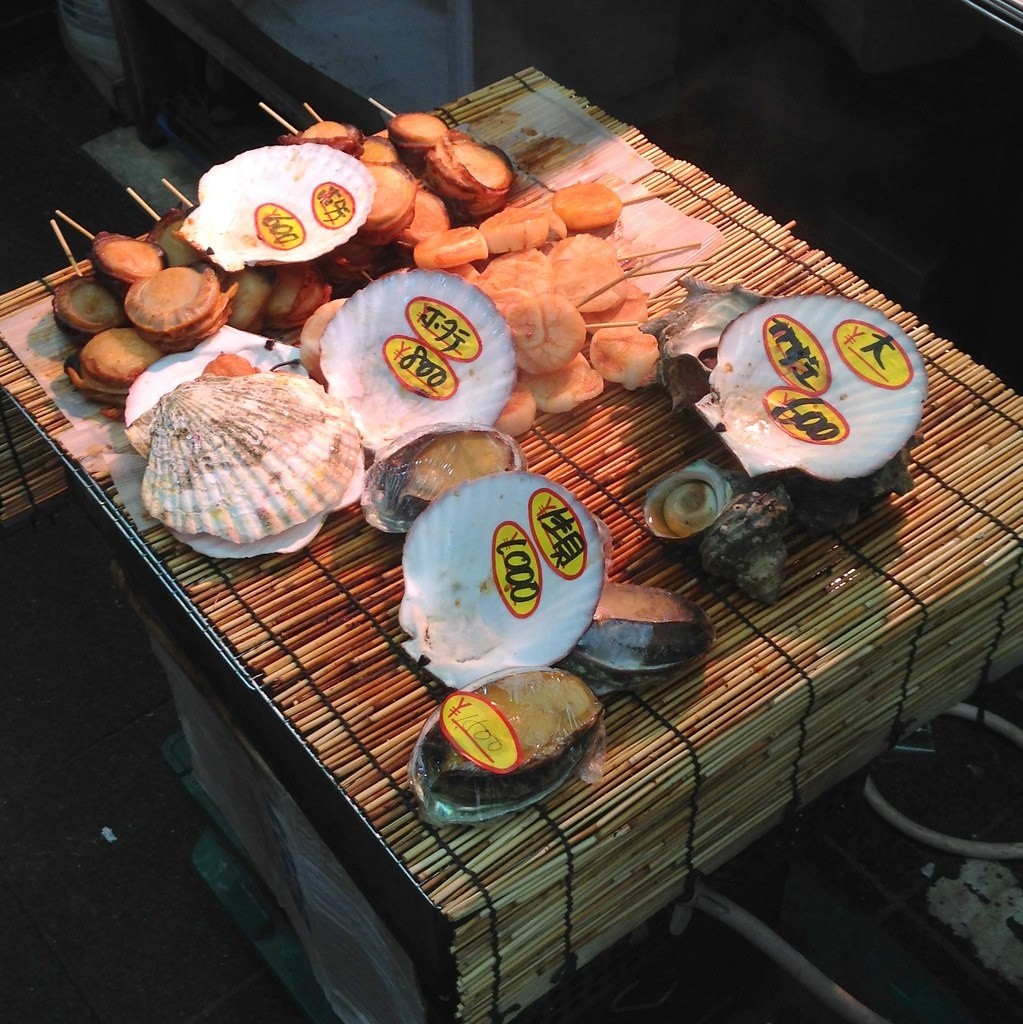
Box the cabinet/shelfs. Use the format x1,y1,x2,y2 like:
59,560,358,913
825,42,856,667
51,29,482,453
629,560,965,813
107,0,679,163
